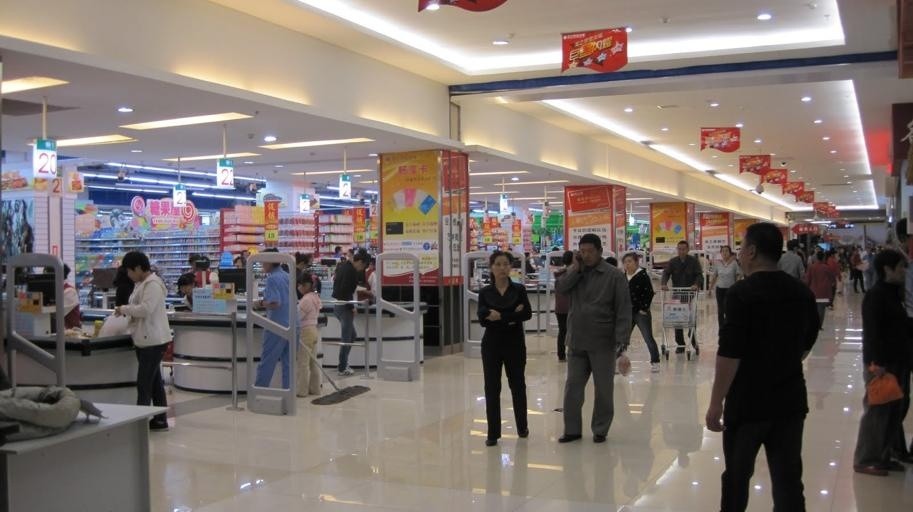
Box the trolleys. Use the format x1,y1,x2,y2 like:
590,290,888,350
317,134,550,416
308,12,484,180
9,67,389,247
654,284,704,360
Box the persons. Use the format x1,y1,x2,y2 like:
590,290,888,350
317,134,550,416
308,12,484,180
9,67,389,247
846,246,881,293
508,246,562,279
551,233,633,443
555,252,572,363
780,241,844,330
707,246,743,345
41,261,79,330
702,220,820,511
699,253,709,291
851,248,912,478
661,240,702,354
476,251,533,447
113,250,173,430
174,244,377,396
606,252,662,373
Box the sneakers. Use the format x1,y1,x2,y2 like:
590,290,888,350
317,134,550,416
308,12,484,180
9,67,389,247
650,362,660,372
338,366,353,375
676,348,685,353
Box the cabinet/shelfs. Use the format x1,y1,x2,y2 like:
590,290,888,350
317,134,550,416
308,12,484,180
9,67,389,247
76,215,354,319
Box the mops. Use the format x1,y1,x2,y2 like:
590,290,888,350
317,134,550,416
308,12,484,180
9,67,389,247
297,340,371,404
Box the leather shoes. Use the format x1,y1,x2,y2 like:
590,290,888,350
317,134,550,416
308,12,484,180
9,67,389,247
517,427,528,437
854,461,906,476
485,440,497,446
558,434,582,442
593,434,606,442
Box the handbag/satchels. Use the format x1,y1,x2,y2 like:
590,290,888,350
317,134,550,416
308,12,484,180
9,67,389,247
867,365,904,406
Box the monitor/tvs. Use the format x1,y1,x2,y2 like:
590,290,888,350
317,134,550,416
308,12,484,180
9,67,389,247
26,273,55,305
219,268,246,294
